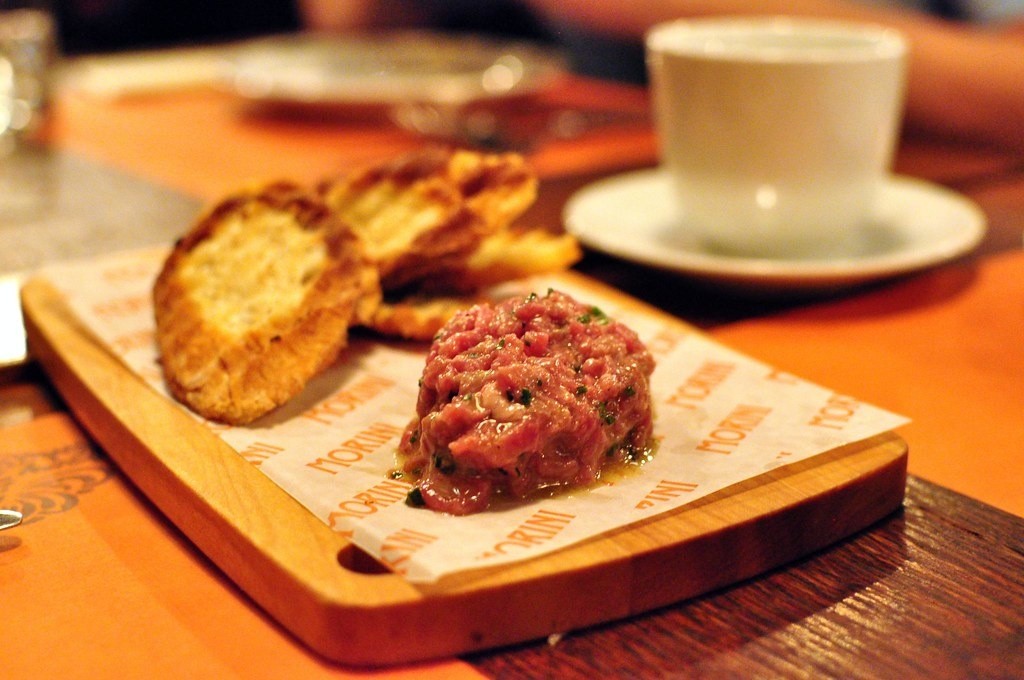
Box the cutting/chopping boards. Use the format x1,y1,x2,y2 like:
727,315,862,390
16,251,914,669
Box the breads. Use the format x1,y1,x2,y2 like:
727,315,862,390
152,147,581,426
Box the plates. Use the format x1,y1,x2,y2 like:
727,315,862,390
559,161,985,290
234,33,565,115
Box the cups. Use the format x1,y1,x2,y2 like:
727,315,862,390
642,12,911,255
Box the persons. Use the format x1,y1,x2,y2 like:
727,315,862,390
526,0,1024,153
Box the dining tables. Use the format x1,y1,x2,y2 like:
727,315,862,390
0,22,1024,680
